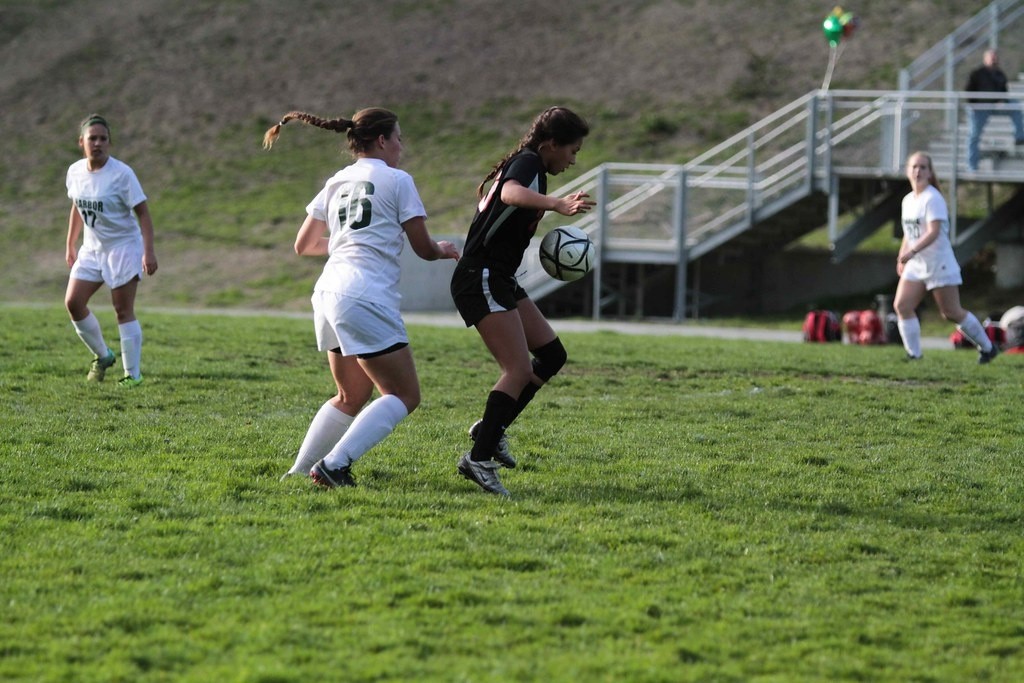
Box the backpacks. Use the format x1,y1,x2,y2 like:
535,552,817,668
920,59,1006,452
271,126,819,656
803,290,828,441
803,311,904,345
953,306,1024,351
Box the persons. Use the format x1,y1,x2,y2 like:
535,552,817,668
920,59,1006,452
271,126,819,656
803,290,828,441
65,115,158,386
451,106,597,495
893,151,1000,365
964,49,1024,173
263,108,461,492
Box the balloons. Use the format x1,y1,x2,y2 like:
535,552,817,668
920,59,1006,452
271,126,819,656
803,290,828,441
824,6,858,46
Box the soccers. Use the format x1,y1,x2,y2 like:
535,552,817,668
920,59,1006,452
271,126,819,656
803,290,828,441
539,225,595,282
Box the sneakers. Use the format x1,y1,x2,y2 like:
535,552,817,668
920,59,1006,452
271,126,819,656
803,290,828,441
117,374,144,389
457,450,510,496
87,350,116,381
311,459,356,489
468,418,516,468
978,344,997,363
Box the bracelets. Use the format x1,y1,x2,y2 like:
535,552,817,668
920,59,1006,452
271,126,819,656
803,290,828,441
910,246,916,255
